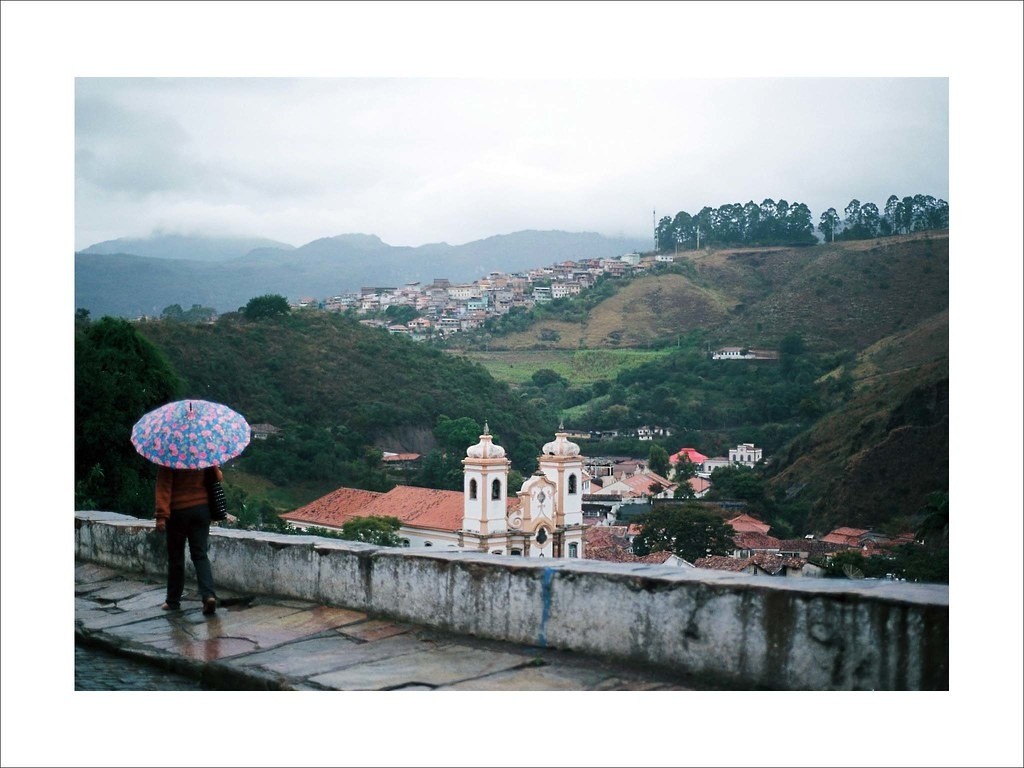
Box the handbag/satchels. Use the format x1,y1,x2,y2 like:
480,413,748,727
208,481,228,521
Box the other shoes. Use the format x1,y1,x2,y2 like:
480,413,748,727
203,597,217,615
161,603,169,610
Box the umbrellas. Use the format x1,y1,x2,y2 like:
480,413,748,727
130,398,251,469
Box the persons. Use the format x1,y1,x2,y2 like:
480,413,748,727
155,463,227,612
